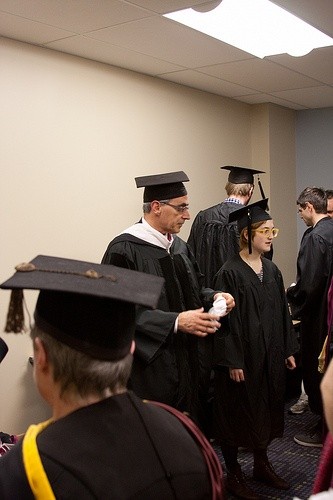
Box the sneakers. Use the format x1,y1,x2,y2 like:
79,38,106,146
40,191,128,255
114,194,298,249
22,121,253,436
253,457,290,489
226,470,258,500
289,395,308,414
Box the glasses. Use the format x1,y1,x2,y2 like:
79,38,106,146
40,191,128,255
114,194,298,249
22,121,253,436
247,227,280,238
158,201,190,215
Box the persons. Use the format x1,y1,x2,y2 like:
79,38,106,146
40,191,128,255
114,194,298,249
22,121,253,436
0,255,224,500
211,198,300,500
188,166,273,290
101,171,235,424
0,338,24,457
286,186,333,447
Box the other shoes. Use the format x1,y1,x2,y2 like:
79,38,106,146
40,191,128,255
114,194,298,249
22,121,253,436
294,432,324,446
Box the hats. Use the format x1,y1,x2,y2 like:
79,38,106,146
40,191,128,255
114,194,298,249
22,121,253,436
1,255,166,365
227,198,272,255
220,165,269,211
135,171,189,203
